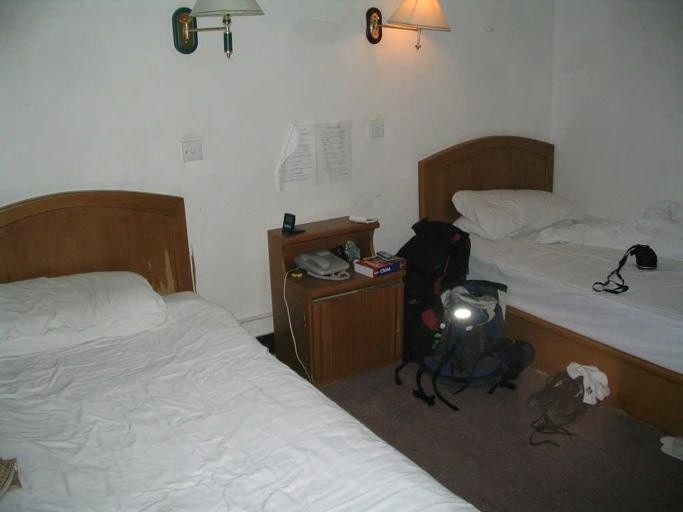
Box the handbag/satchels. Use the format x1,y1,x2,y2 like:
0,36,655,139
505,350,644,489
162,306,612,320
1,457,24,497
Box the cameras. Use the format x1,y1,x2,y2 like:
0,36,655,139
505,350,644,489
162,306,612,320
635,245,658,269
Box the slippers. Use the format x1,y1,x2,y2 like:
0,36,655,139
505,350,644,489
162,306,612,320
660,444,682,462
659,435,682,446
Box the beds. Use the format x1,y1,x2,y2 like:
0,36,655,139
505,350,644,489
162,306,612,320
416,134,682,440
0,189,487,512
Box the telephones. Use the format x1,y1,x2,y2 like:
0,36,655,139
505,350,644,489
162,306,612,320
294,249,350,276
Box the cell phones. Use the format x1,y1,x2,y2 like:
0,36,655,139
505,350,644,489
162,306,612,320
376,250,391,260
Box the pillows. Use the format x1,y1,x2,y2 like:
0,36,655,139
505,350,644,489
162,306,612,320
452,216,532,242
0,268,168,360
450,188,585,240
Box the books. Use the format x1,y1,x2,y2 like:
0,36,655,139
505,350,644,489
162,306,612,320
352,255,408,279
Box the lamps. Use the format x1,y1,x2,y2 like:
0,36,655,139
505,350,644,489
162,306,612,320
365,1,452,52
170,0,265,60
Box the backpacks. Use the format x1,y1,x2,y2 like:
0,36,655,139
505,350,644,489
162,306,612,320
394,217,471,385
412,279,534,410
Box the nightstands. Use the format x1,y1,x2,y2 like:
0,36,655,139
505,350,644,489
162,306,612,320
266,216,407,388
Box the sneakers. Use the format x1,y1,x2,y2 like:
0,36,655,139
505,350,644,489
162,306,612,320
544,376,583,416
527,376,572,410
